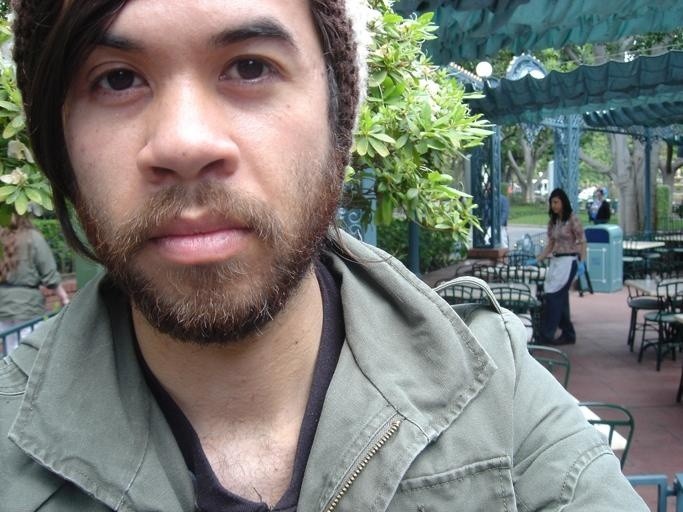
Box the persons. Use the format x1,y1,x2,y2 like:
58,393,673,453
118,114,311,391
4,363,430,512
523,186,588,348
0,210,71,358
482,180,511,251
585,187,612,225
0,0,657,512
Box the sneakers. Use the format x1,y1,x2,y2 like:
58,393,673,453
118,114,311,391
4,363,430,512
551,336,573,346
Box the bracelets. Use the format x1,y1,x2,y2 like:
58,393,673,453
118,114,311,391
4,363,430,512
61,298,70,305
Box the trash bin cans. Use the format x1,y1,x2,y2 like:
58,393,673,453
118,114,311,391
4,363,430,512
579,224,623,293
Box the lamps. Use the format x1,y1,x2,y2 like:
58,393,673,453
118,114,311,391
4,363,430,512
476,62,492,78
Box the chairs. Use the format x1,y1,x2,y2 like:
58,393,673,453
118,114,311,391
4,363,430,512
437,228,683,472
